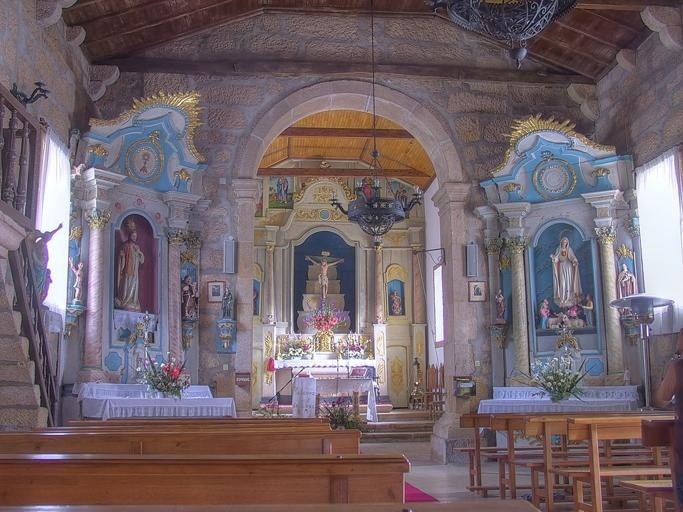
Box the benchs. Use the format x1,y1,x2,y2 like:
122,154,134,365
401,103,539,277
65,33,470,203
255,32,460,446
1,419,536,511
459,408,683,512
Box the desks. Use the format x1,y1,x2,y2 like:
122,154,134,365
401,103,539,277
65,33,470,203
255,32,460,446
84,397,234,420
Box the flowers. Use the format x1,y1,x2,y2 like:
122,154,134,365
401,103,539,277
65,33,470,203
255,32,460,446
134,345,194,401
306,300,340,335
516,350,597,402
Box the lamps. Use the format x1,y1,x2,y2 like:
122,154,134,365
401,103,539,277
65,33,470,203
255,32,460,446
329,0,423,248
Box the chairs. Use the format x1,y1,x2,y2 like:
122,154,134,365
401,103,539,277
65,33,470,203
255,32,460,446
417,363,447,418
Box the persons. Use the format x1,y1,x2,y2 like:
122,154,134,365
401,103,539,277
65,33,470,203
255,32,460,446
68,254,85,300
221,287,232,319
389,290,401,315
494,288,506,320
539,299,550,330
304,253,345,302
656,329,683,512
181,275,199,316
114,230,145,312
616,263,638,299
549,237,580,303
578,294,595,326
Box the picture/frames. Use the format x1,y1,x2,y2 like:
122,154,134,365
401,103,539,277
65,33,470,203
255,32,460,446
469,280,487,302
206,280,227,304
